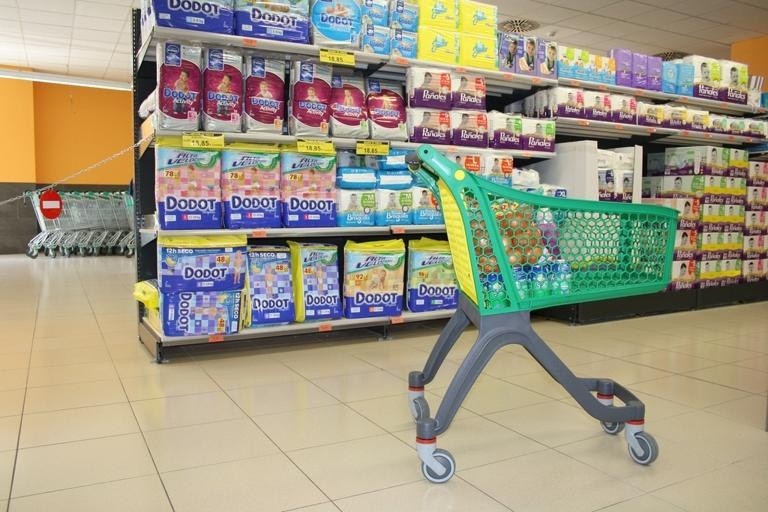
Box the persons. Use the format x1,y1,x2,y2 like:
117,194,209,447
556,85,636,120
729,68,740,89
161,39,556,316
700,62,713,86
595,139,768,291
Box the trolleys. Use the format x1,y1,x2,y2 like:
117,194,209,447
406,144,680,484
23,189,138,259
